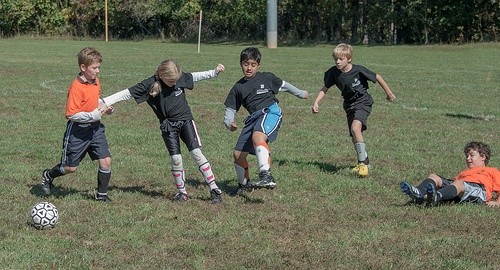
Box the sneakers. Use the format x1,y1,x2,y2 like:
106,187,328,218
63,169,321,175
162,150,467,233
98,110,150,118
41,169,55,195
400,181,424,205
426,183,438,207
92,188,112,203
352,150,370,176
248,170,276,190
229,179,253,197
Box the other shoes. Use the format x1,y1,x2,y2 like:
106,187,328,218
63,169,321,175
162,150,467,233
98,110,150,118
210,188,223,204
171,191,188,202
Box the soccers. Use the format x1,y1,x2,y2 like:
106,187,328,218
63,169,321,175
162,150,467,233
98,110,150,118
30,201,58,231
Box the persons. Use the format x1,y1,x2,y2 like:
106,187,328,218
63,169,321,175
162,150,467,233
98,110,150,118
99,59,225,204
223,47,309,197
312,43,396,178
399,141,500,209
41,47,114,203
